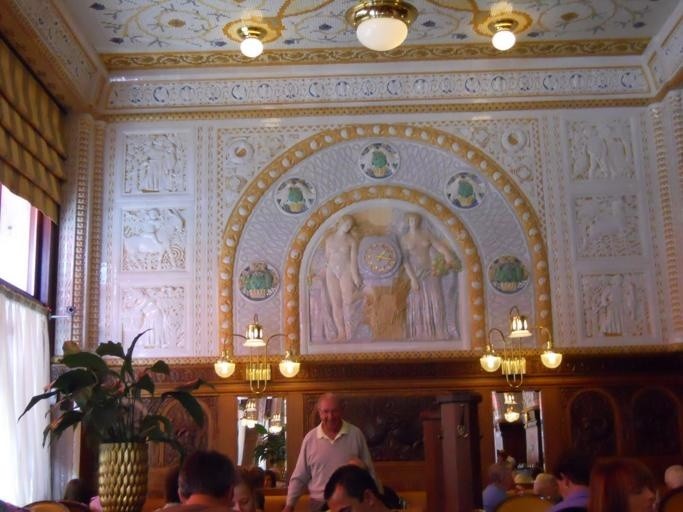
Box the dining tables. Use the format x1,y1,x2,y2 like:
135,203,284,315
16,330,217,510
250,421,285,477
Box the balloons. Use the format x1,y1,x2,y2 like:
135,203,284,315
357,234,404,281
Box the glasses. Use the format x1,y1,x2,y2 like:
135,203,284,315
477,304,562,389
502,392,520,424
235,1,518,60
212,313,302,392
243,397,281,433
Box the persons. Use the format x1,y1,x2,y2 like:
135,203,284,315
478,461,514,512
580,124,611,181
136,133,177,191
583,452,657,512
322,464,393,512
321,214,363,343
543,448,594,512
278,391,389,512
137,205,165,248
258,470,277,489
400,212,458,341
594,275,636,337
155,448,241,512
129,286,167,350
153,467,184,512
230,467,264,512
60,475,88,506
662,464,682,498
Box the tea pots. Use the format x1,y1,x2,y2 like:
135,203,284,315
493,389,542,476
235,396,287,484
